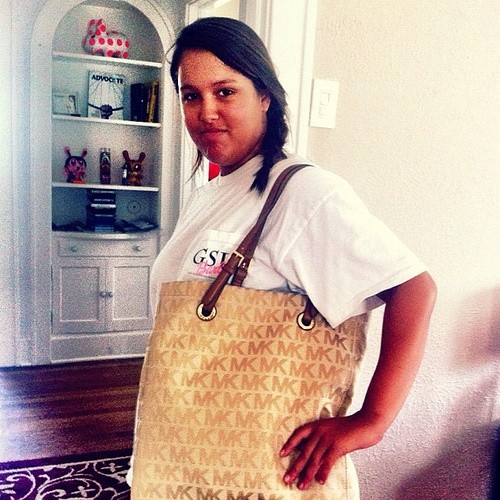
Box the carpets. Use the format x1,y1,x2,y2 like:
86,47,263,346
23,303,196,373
0,447,133,500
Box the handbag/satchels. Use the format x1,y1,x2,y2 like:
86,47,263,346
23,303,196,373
130,280,370,500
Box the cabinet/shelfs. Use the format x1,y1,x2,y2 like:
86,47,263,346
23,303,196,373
30,0,180,366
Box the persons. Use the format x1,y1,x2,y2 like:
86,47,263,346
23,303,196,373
124,17,436,499
